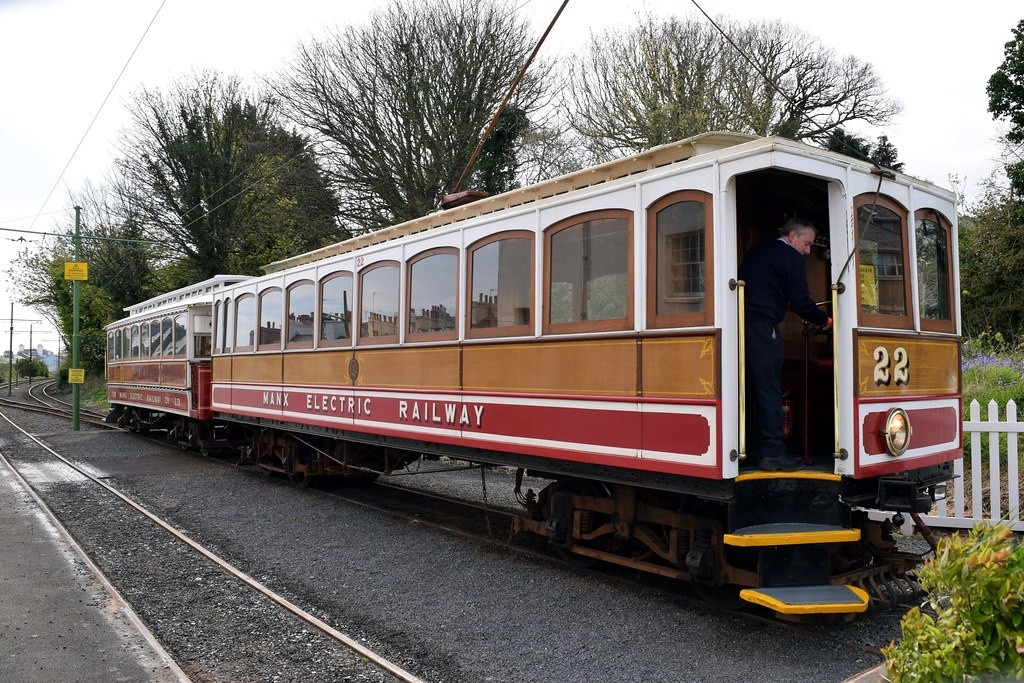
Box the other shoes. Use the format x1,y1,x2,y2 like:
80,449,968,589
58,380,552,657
746,450,762,468
761,452,805,471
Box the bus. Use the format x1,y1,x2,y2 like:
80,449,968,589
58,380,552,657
104,131,963,624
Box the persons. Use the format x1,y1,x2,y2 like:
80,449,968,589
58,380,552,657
737,214,832,472
